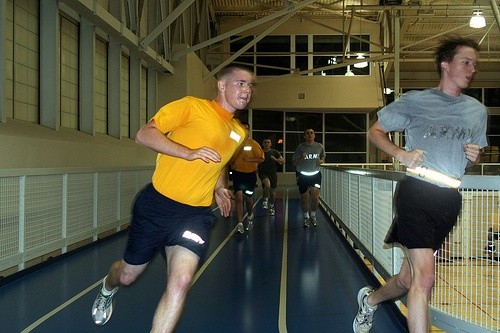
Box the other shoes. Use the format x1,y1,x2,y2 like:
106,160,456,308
311,219,317,226
236,225,244,233
246,219,253,230
304,218,310,226
270,209,274,216
262,202,268,209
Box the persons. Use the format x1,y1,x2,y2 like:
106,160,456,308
258,138,285,216
230,124,265,234
292,128,326,226
352,36,488,333
91,68,252,333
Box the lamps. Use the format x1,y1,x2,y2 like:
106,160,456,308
345,45,354,76
354,9,368,68
469,7,486,28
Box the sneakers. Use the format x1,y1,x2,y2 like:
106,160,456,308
353,287,378,333
92,283,120,327
